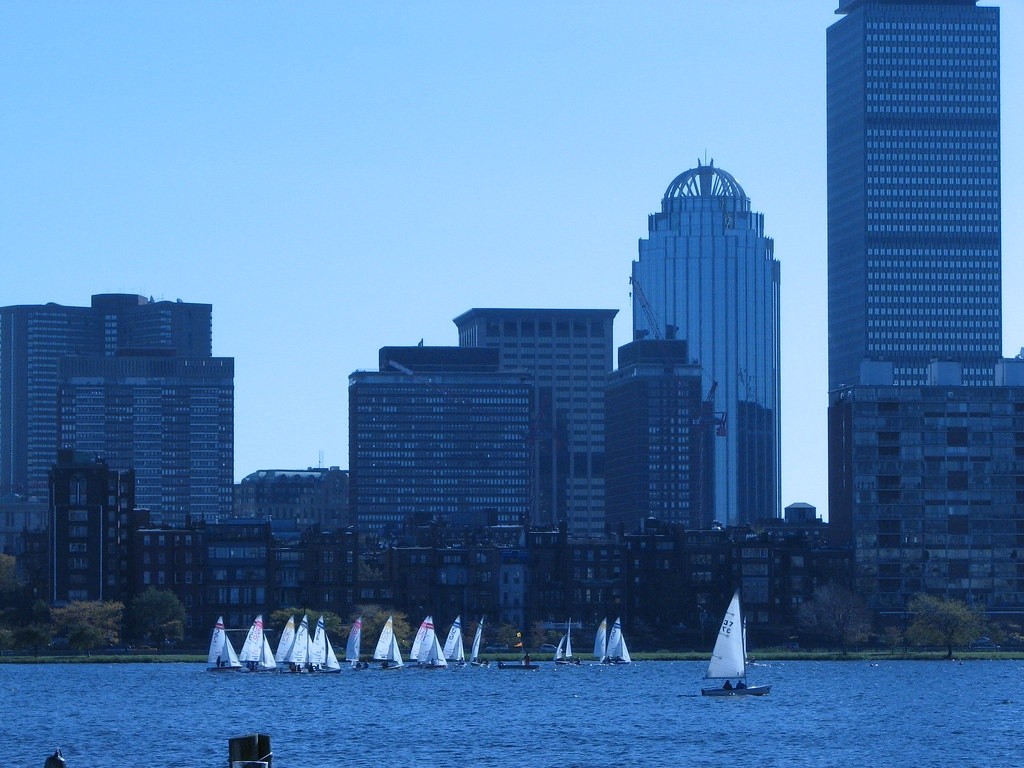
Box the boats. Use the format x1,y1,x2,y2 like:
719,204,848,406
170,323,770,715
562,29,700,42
498,661,540,670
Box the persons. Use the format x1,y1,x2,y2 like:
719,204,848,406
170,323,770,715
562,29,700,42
602,656,611,663
524,653,530,666
472,656,489,664
459,658,465,664
356,663,361,668
576,656,581,663
246,661,259,670
380,660,388,668
736,680,746,689
216,656,222,667
363,660,369,667
723,680,732,690
288,663,314,673
569,657,574,663
751,656,755,663
431,658,434,666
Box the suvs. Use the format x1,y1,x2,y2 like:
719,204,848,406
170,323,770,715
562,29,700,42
484,642,509,653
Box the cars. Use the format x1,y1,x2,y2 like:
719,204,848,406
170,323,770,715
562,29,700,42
967,637,995,646
133,646,158,654
540,644,563,654
48,638,70,650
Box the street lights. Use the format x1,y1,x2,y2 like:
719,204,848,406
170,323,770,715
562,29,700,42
903,593,909,653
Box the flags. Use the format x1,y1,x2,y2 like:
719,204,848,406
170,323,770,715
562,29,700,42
514,641,522,647
516,632,521,637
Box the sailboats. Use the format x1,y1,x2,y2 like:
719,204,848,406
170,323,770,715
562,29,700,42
592,616,607,666
307,615,342,674
284,615,314,674
371,616,405,671
607,617,631,666
206,615,243,672
553,633,570,665
411,615,448,669
442,615,468,669
344,615,363,671
702,588,774,699
564,617,583,665
469,613,490,668
256,632,277,673
237,615,264,672
